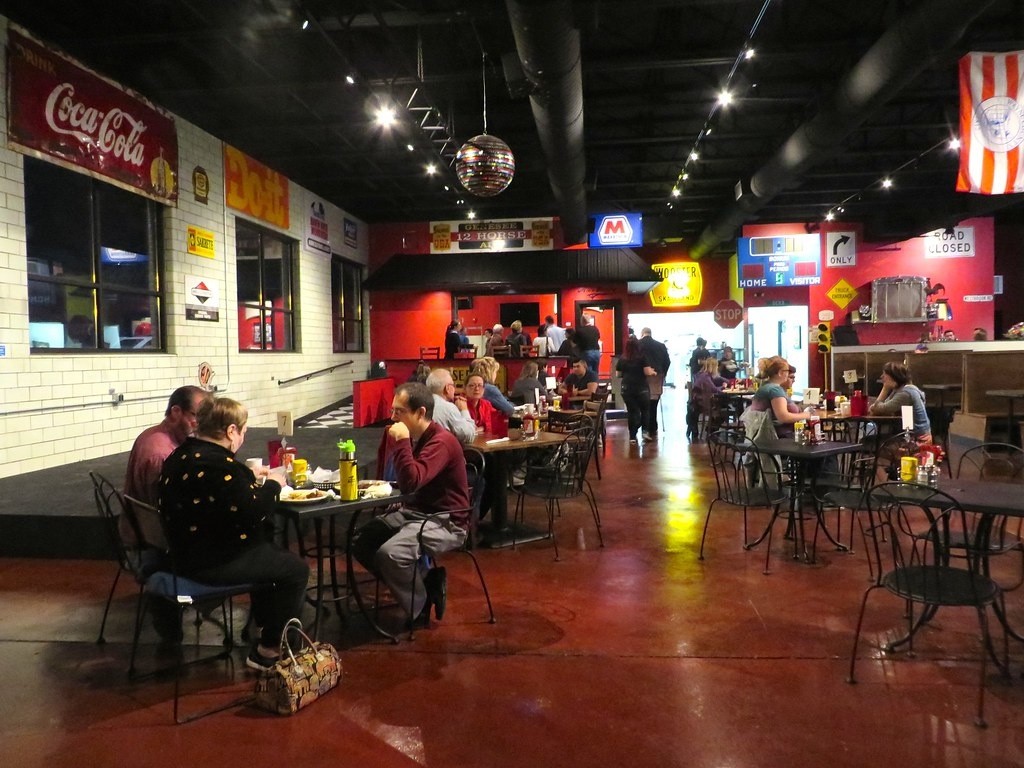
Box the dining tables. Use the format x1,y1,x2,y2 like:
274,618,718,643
273,487,418,643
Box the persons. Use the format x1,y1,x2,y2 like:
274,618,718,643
615,328,670,442
944,330,955,340
974,328,986,340
748,355,844,510
445,320,480,358
559,359,600,408
355,383,469,626
533,316,600,373
484,320,531,357
689,339,744,438
426,357,517,541
510,362,555,404
537,358,551,387
859,362,933,462
156,397,309,670
125,386,212,643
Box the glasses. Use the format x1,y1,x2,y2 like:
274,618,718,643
782,369,792,373
448,383,456,388
390,408,407,415
469,383,484,388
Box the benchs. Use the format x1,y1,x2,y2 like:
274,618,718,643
968,412,1024,450
926,403,961,409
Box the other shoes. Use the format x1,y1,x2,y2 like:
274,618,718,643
498,525,519,537
642,430,653,441
629,436,638,443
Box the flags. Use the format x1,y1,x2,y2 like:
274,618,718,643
956,51,1024,194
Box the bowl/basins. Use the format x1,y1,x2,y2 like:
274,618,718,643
834,408,841,413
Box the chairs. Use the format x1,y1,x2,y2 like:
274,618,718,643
86,345,1024,728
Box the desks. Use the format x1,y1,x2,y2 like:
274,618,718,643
468,431,585,549
871,478,1024,686
732,438,863,559
539,395,591,403
722,388,756,413
810,408,902,481
508,409,598,422
922,383,962,409
985,390,1024,453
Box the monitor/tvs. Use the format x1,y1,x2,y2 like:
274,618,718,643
500,302,540,327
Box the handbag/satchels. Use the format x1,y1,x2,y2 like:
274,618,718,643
256,620,344,716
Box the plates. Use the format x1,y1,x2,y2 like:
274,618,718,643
333,479,387,491
278,489,328,504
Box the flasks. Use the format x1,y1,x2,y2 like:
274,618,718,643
337,440,360,501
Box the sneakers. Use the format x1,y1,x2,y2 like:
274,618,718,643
246,645,285,671
424,566,447,621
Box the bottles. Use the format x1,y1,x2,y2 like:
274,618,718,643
855,390,862,396
918,456,942,489
799,426,811,443
839,396,845,407
159,148,167,197
729,376,759,391
811,416,821,442
507,377,569,442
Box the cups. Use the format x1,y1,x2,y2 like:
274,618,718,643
851,395,868,416
840,402,851,415
244,458,263,467
794,423,804,443
901,457,918,481
291,459,307,488
